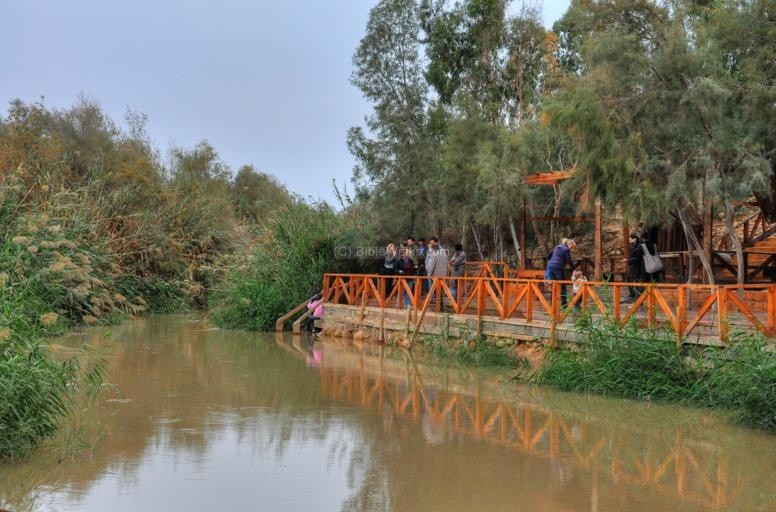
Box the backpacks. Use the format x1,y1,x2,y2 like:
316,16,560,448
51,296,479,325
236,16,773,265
641,243,664,274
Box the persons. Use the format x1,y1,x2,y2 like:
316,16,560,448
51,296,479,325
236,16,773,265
303,295,323,332
619,231,643,304
542,236,577,310
380,237,467,310
568,259,589,310
302,337,322,373
634,232,662,306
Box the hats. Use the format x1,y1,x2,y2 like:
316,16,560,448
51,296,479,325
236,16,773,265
455,243,462,250
